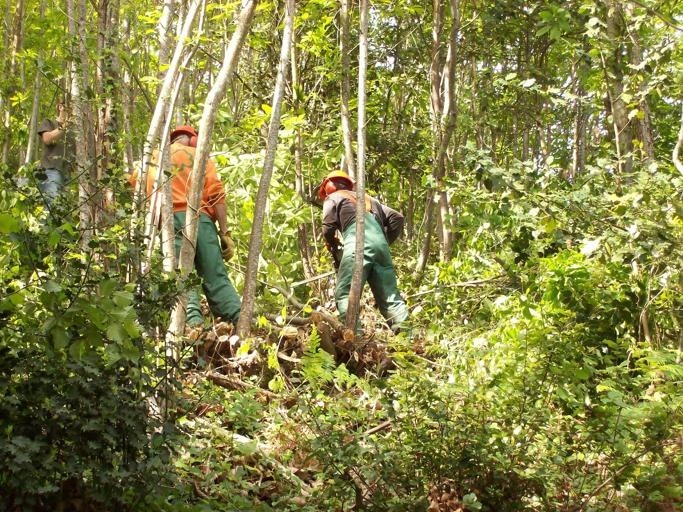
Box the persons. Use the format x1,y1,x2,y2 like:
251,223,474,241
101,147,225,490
319,170,413,342
127,125,242,347
35,97,74,229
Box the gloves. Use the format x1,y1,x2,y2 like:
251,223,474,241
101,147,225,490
218,226,235,263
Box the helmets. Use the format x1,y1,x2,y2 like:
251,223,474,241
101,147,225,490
318,171,354,200
170,124,198,140
